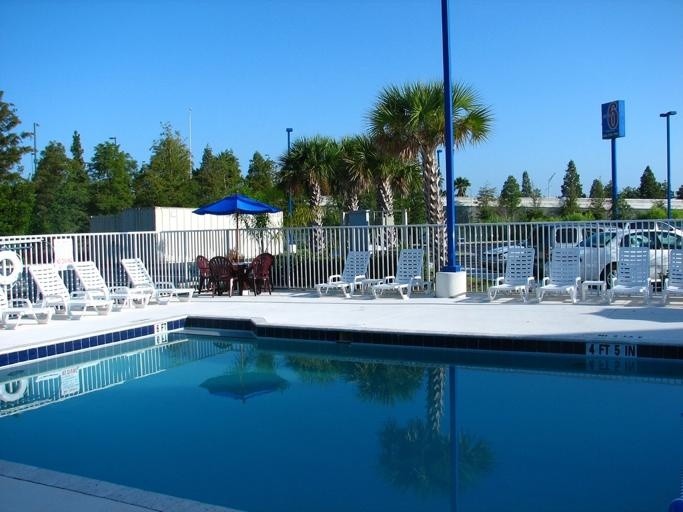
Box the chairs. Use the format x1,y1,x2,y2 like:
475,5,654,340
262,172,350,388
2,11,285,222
315,251,370,298
486,247,534,304
608,247,652,304
196,253,274,296
660,248,683,305
536,247,582,304
0,258,195,330
372,249,432,300
61,371,80,396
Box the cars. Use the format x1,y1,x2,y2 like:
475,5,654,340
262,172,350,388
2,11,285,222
479,221,682,291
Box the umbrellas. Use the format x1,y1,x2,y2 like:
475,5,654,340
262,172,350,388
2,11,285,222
190,189,282,262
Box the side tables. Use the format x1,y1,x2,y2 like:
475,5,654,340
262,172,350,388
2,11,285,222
582,281,606,302
361,278,381,295
